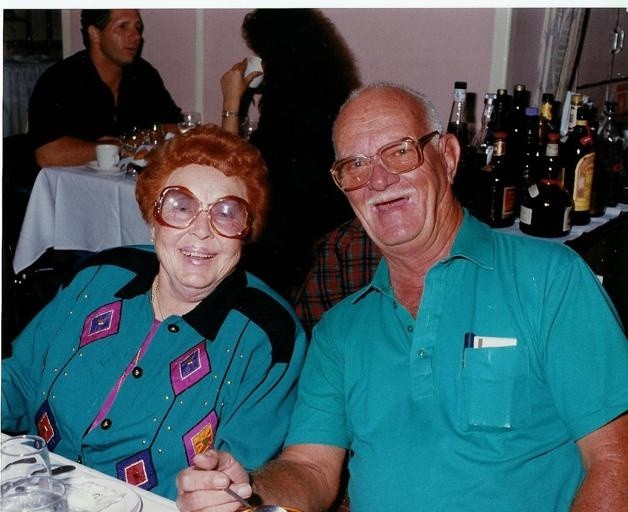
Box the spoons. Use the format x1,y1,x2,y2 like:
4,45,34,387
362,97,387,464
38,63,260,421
194,465,287,512
127,165,138,177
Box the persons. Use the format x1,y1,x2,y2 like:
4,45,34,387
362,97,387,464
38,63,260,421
219,9,362,297
177,80,628,512
1,122,310,503
25,10,185,275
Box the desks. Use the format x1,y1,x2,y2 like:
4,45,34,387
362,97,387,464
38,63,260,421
464,202,628,330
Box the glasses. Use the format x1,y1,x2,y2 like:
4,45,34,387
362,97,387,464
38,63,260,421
330,130,440,192
152,185,254,240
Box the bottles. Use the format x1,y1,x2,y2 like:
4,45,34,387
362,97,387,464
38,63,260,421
510,83,529,137
463,91,478,143
529,117,551,181
566,93,582,132
519,134,575,238
479,132,514,229
492,89,511,144
520,109,534,181
604,100,622,211
565,106,600,228
588,110,606,218
472,92,498,149
538,93,556,148
443,82,472,207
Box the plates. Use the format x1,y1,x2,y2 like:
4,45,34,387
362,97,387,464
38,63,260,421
86,160,128,175
61,473,144,512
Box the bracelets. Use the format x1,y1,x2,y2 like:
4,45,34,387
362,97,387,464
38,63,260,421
222,108,239,121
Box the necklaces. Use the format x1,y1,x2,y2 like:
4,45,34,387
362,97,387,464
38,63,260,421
151,276,166,323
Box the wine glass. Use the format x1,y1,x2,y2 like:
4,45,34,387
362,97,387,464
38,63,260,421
145,122,166,146
240,118,259,138
1,435,52,490
178,104,201,134
120,125,145,154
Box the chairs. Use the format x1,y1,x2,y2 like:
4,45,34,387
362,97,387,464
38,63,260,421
2,132,77,353
288,218,384,330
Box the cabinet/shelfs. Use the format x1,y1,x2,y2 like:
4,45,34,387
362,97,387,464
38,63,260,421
497,8,628,127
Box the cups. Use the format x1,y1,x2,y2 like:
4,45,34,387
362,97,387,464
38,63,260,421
0,474,68,511
96,144,120,167
244,57,264,89
